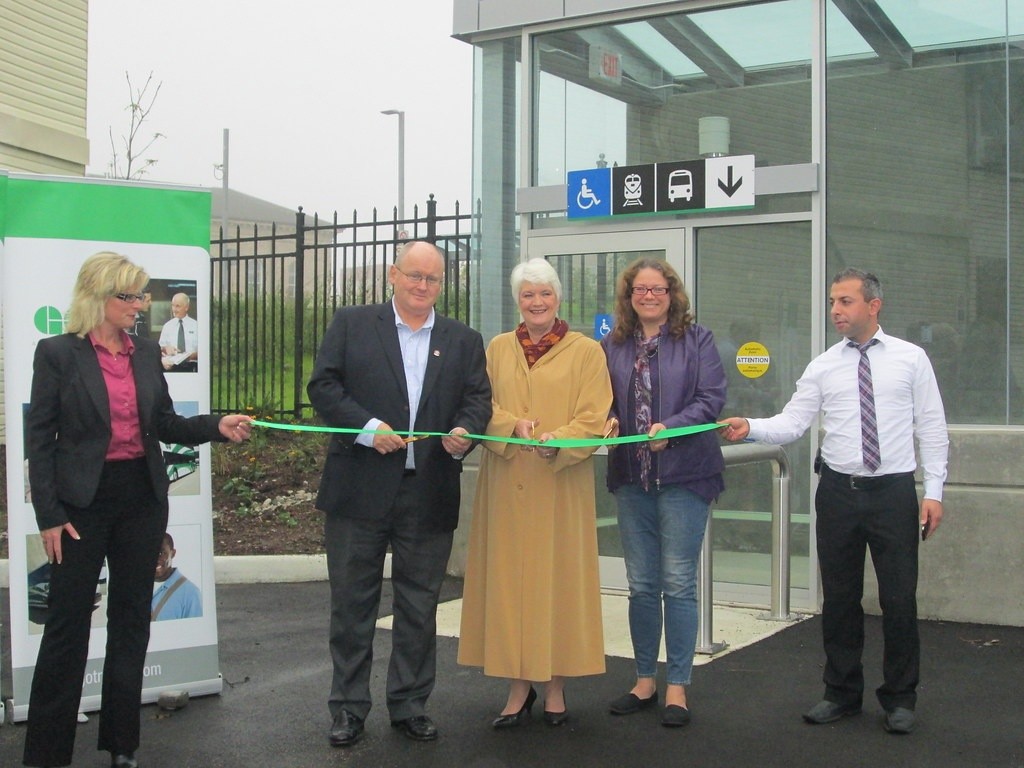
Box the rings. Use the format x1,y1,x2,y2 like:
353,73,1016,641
545,453,550,458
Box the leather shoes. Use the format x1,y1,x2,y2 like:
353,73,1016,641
801,699,862,723
392,715,439,741
329,709,365,746
112,751,137,768
882,706,916,735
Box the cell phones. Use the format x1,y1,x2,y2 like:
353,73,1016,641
921,521,930,541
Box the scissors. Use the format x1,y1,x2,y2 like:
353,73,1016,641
529,421,536,453
402,434,429,442
602,416,619,450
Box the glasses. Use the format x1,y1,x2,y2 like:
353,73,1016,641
115,293,146,303
629,286,670,296
397,267,443,287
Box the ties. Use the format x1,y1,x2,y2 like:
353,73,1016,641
178,320,185,353
848,339,881,473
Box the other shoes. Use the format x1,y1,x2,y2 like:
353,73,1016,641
661,699,690,726
608,689,659,714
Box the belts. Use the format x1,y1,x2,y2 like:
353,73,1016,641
821,464,913,490
404,469,416,477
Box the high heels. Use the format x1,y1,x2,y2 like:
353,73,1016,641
543,689,568,726
492,683,537,728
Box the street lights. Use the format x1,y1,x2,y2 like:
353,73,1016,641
382,109,406,234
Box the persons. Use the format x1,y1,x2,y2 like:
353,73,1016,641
456,257,614,728
150,533,202,621
717,266,948,733
306,242,492,744
22,251,255,768
124,287,198,372
601,258,728,728
